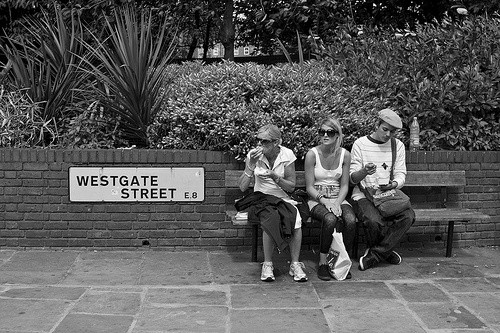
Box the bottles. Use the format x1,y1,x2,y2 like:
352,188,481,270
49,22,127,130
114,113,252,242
410,116,419,152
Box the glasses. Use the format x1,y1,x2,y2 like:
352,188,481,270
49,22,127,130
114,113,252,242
317,128,339,138
255,137,277,144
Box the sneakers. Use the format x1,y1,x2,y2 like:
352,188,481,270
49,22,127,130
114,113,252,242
260,262,275,281
289,262,308,281
318,262,331,279
360,248,377,270
385,251,401,264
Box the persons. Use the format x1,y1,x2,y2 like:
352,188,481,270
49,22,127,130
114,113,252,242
304,117,356,280
350,109,416,271
238,124,308,282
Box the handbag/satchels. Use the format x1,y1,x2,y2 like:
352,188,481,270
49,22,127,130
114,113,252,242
290,193,309,225
363,185,411,217
326,228,353,280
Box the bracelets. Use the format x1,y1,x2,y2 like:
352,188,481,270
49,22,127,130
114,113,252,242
247,164,254,172
275,177,279,183
316,194,322,201
244,171,253,178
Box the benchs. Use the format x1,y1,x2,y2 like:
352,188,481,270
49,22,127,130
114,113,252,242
224,169,491,261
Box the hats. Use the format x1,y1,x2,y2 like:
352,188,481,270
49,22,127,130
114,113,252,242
378,109,403,129
